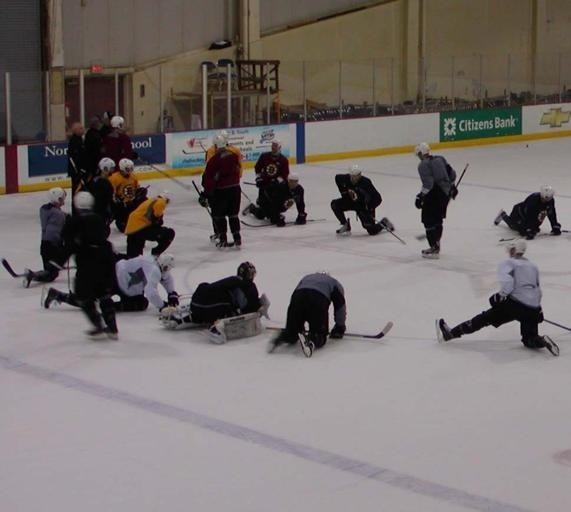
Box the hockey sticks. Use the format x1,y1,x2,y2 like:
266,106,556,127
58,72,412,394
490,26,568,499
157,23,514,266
265,321,393,339
1,259,77,277
139,158,191,189
355,213,406,246
182,150,206,154
192,181,234,248
499,230,571,241
48,259,76,270
240,219,326,228
415,163,470,241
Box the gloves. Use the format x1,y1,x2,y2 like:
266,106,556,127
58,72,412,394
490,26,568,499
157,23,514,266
295,212,307,225
276,215,286,226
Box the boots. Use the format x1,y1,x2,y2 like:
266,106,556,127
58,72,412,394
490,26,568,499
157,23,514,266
83,328,106,340
436,317,462,344
419,241,440,259
202,327,224,345
214,234,228,249
157,313,179,330
242,202,256,216
233,232,242,247
380,216,395,231
298,333,316,357
103,327,119,340
207,232,218,242
494,208,506,225
266,327,286,353
40,285,56,310
540,334,560,356
337,218,352,236
22,266,35,288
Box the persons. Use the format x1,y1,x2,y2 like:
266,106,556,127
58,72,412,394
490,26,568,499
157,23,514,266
494,186,560,240
330,163,394,236
414,143,457,255
24,187,179,340
67,116,138,217
267,268,346,357
197,128,307,252
160,261,272,345
80,157,175,261
439,239,560,357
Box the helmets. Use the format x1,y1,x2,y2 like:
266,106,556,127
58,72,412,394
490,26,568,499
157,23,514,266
48,186,69,205
159,190,177,202
316,267,331,278
349,164,363,178
271,138,284,154
157,254,176,272
119,158,135,178
213,134,228,149
219,130,229,143
540,184,555,202
110,116,125,130
236,262,257,282
503,239,527,255
287,173,300,185
74,192,95,212
99,157,116,175
414,141,431,159
